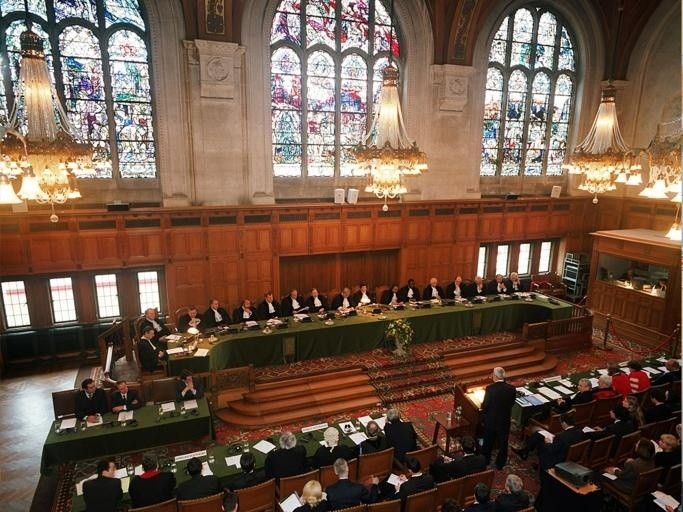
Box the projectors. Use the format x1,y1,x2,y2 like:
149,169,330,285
554,460,593,489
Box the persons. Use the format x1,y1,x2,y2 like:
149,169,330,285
85,366,683,511
75,368,198,424
138,274,524,372
659,278,667,291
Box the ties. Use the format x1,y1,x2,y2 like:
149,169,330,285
88,395,92,400
123,395,126,402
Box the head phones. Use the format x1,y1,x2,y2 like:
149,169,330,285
182,467,190,476
227,444,243,455
59,428,73,437
190,410,200,417
344,424,352,432
128,419,138,427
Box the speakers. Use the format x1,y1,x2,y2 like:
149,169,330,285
334,188,345,203
347,189,359,204
12,198,29,213
551,186,562,198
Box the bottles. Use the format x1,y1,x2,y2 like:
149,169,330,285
446,406,462,421
243,436,249,453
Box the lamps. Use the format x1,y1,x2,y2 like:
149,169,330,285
570,1,634,201
0,0,94,223
619,86,682,242
349,0,429,216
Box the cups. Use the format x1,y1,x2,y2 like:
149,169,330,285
208,455,215,464
355,421,360,430
126,464,134,477
170,463,177,473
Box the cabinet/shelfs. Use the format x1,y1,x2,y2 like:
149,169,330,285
561,252,593,297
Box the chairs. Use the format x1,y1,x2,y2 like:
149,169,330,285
428,352,681,509
72,410,496,512
38,374,224,463
129,274,598,373
